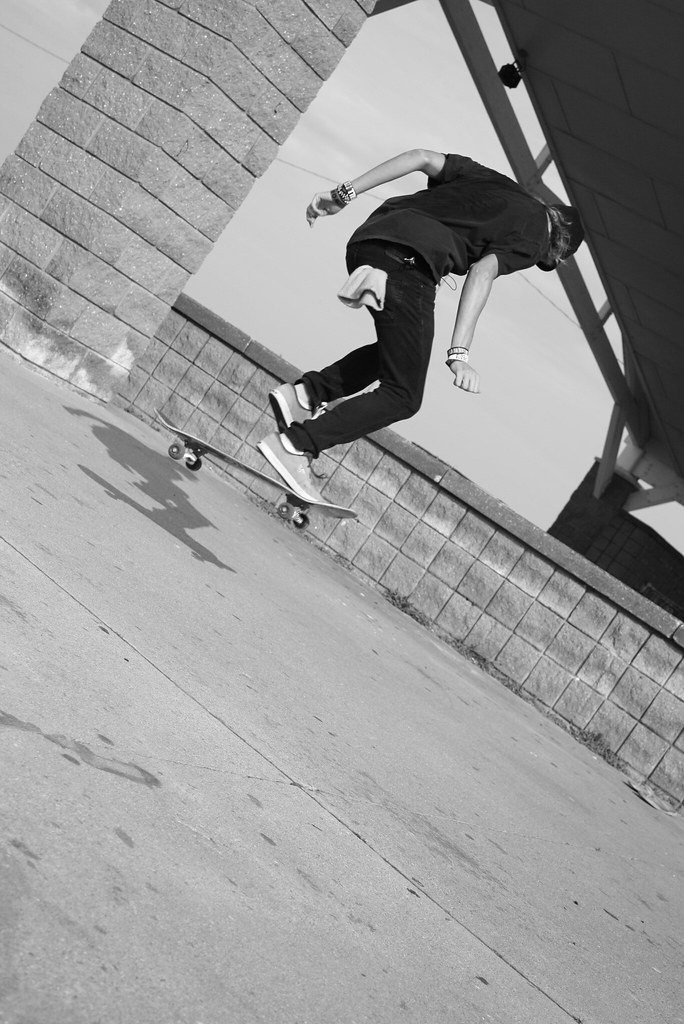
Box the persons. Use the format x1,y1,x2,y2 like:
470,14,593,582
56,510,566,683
257,146,586,508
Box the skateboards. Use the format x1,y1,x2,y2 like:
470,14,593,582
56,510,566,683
152,406,359,530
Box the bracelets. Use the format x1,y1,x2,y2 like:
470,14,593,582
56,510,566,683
331,189,347,208
447,346,469,356
449,354,468,363
337,184,351,204
343,180,357,199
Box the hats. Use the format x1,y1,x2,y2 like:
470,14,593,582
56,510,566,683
536,204,584,271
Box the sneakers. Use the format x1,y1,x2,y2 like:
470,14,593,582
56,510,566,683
257,433,323,500
269,382,317,435
